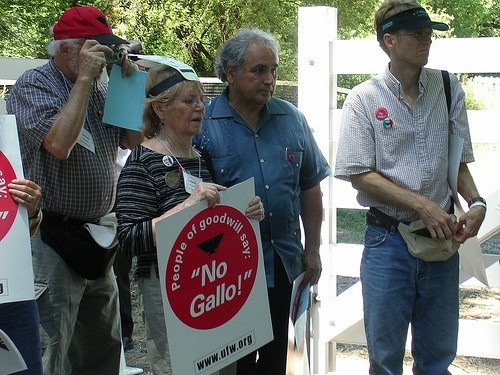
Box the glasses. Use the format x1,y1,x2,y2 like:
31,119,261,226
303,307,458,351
395,31,438,40
171,96,211,106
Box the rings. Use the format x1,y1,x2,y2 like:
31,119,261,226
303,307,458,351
27,195,33,202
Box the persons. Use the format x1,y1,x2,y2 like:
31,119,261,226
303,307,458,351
5,8,149,375
116,65,265,375
0,179,43,375
192,30,332,375
334,0,487,375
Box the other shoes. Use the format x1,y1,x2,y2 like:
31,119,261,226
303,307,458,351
123,337,134,351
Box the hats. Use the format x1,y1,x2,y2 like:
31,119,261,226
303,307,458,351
378,7,449,37
53,7,130,46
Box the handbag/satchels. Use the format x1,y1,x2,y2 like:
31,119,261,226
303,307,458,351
40,210,120,278
370,198,461,262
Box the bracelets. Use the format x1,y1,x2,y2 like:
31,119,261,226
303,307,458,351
470,202,486,212
468,197,486,207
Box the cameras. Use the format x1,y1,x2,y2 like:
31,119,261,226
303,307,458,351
104,46,124,64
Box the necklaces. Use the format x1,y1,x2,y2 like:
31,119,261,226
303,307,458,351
153,132,200,177
